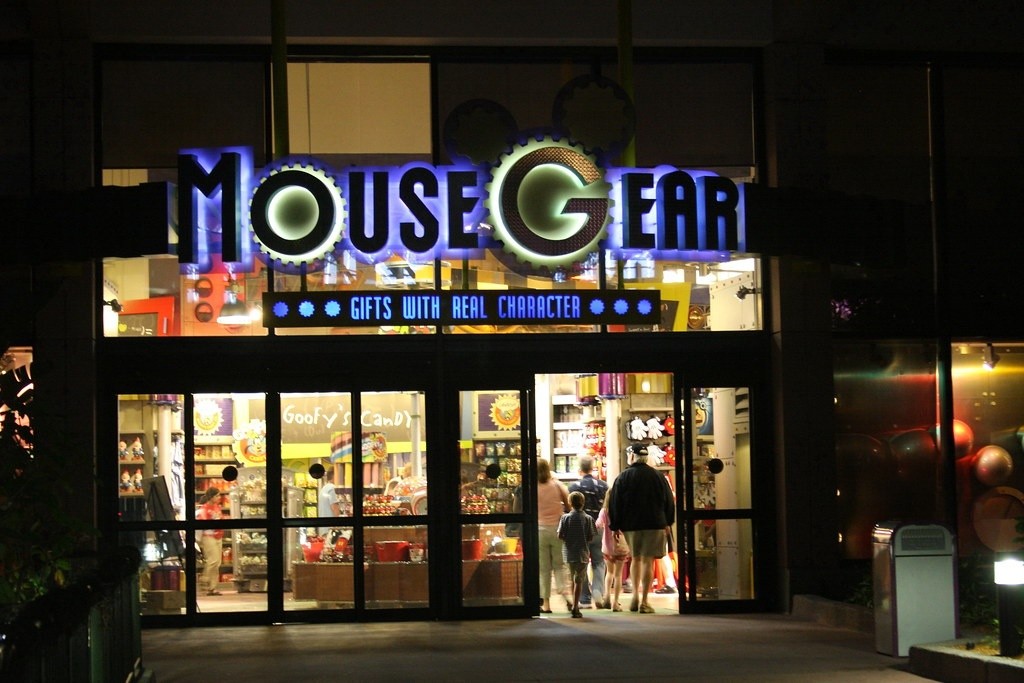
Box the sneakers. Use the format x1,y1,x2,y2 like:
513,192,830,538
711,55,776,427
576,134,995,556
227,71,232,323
640,603,655,613
630,600,639,612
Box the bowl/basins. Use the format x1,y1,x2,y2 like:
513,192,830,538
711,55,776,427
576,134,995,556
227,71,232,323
462,539,481,561
376,541,409,562
302,542,323,562
493,537,518,554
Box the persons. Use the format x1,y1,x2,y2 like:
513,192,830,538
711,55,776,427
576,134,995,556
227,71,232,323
608,442,675,612
610,528,679,595
566,454,609,608
384,461,412,498
316,465,340,536
195,487,224,595
537,458,572,613
594,486,630,612
460,463,501,489
555,490,598,618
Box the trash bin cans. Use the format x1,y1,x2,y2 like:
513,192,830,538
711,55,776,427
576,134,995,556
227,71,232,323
870,519,961,658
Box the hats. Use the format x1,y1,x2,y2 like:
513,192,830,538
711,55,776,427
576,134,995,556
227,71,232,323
625,444,650,456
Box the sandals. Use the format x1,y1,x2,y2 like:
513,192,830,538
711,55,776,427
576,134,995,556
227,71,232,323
206,589,223,596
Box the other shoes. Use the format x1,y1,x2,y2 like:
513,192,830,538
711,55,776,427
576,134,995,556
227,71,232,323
571,608,583,618
539,606,552,613
655,585,676,594
622,582,633,593
594,601,611,610
566,603,573,611
613,604,624,611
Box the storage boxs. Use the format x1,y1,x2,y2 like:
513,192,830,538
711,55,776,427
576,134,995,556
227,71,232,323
239,577,268,591
140,589,186,615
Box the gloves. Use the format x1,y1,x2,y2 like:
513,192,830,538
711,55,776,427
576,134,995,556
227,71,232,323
645,444,667,467
625,416,649,440
647,417,666,439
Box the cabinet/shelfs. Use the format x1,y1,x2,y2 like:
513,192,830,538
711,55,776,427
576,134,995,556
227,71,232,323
117,394,185,498
292,500,524,602
230,465,304,592
194,434,245,590
550,394,718,551
300,485,318,535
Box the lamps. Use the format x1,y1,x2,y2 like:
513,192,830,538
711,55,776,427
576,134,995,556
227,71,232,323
983,342,1001,371
736,287,753,298
994,550,1024,655
107,298,121,311
